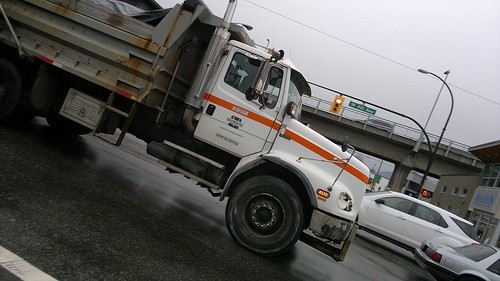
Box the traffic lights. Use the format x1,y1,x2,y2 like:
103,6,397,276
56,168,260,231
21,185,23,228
422,188,433,199
329,96,345,114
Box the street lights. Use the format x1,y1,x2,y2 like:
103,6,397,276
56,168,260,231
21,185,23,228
414,68,454,198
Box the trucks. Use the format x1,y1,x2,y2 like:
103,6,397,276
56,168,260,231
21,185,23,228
0,0,371,262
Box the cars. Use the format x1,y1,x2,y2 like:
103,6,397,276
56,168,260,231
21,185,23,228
413,239,500,281
354,191,480,255
